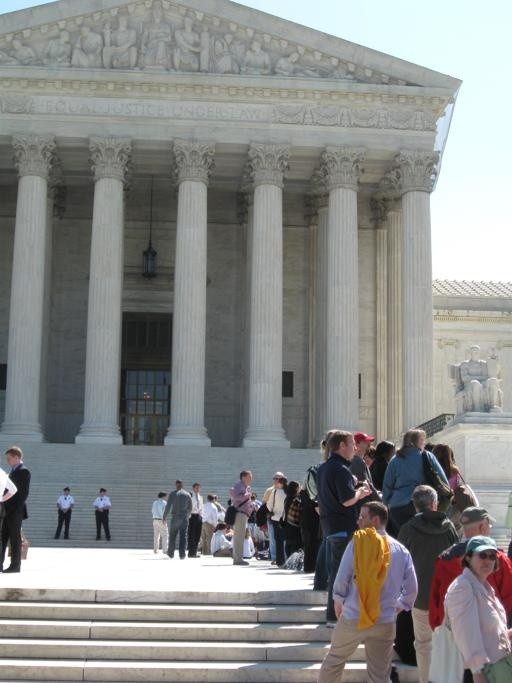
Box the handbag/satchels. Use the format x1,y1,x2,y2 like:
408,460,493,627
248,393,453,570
422,449,456,511
286,495,301,527
224,505,236,524
454,467,479,511
427,624,466,682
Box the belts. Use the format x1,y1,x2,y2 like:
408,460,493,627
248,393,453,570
154,517,161,519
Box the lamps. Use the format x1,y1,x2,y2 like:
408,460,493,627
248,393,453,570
141,174,157,280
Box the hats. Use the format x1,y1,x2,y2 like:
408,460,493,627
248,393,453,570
466,535,499,556
353,431,375,443
460,506,497,524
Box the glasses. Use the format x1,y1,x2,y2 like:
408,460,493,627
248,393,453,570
480,551,496,560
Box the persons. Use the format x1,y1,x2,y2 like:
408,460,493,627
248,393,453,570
456,343,504,412
3,445,30,572
0,0,324,79
0,466,18,571
152,471,322,574
54,487,75,539
92,487,110,540
305,429,512,682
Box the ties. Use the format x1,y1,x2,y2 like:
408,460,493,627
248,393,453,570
196,493,199,508
